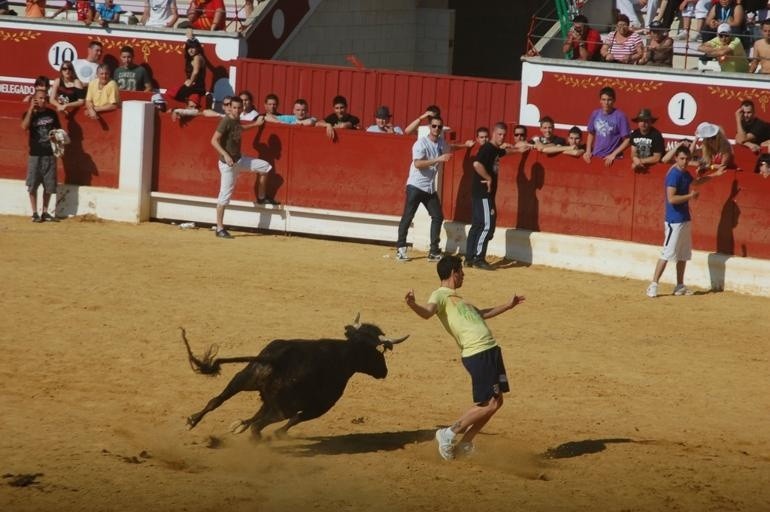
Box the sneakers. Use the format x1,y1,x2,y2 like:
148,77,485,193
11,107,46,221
42,213,59,222
213,226,234,239
476,259,489,266
426,251,447,262
647,282,662,299
256,197,282,208
394,249,411,261
673,284,694,297
435,426,457,460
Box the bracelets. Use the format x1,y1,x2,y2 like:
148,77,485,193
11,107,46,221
417,117,422,122
579,44,588,48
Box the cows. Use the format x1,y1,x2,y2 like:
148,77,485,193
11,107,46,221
176,313,411,444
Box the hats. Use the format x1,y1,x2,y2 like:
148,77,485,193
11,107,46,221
717,24,731,33
647,21,664,31
152,94,167,104
632,110,658,122
373,105,392,118
695,122,719,139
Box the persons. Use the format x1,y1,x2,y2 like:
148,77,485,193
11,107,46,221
677,0,720,42
71,41,103,85
646,145,700,298
463,121,530,270
661,137,703,167
366,105,404,136
44,1,96,26
694,122,733,172
186,92,202,110
94,0,122,24
49,61,85,115
85,63,118,119
749,19,770,74
404,256,526,462
211,96,281,238
23,76,52,110
178,0,226,32
257,94,282,122
314,95,359,140
172,95,234,121
631,108,663,169
138,0,178,29
150,93,167,113
542,126,585,157
396,116,477,262
614,0,669,31
638,20,674,69
264,99,317,127
701,0,745,44
562,16,602,60
735,100,770,151
237,91,260,122
600,15,644,64
241,1,256,23
527,117,565,151
405,105,450,141
583,86,630,167
24,0,46,20
697,22,749,76
1,0,18,16
113,47,153,93
468,127,490,162
506,125,531,152
21,89,61,224
753,153,770,176
174,38,207,101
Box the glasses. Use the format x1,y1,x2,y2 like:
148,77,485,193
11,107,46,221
514,133,524,137
62,66,73,71
431,124,443,129
719,34,730,37
222,102,232,106
